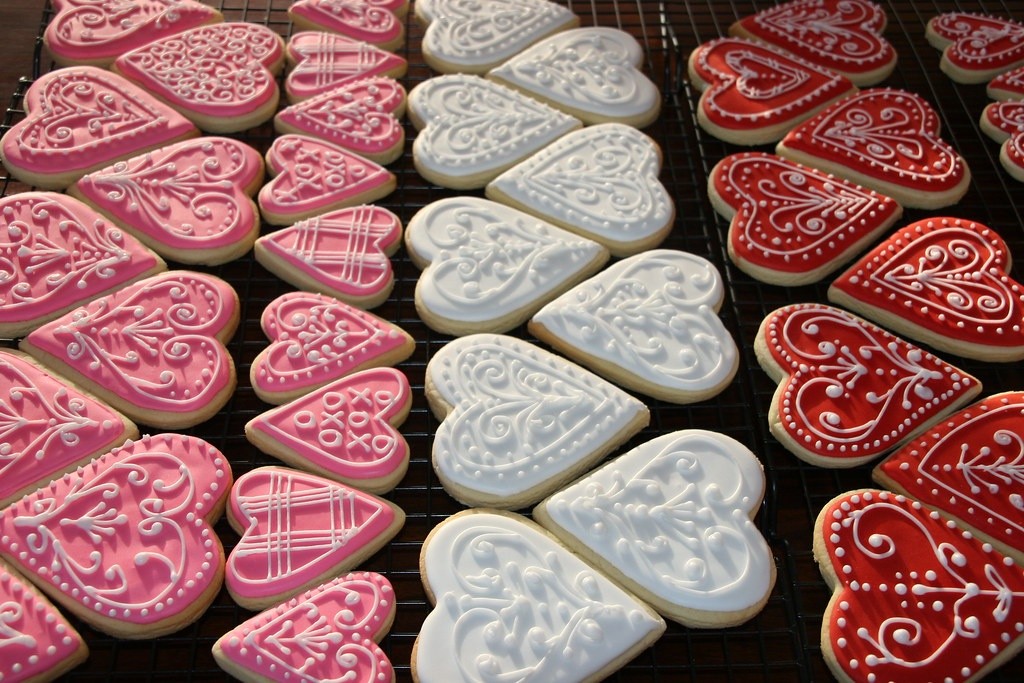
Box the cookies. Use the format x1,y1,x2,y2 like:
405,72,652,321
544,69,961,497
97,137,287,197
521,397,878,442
0,0,1024,683
